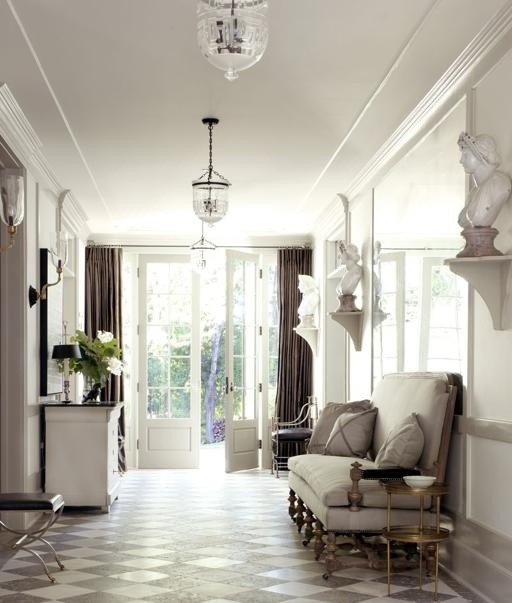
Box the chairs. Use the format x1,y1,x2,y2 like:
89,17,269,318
271,395,319,476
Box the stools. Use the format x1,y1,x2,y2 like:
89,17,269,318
380,479,451,600
0,493,67,583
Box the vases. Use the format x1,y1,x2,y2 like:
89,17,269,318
82,382,102,404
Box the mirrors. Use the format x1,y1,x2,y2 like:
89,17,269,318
365,93,475,418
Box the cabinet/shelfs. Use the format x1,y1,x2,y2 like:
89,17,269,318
42,401,125,513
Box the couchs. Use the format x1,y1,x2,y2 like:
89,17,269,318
286,371,462,581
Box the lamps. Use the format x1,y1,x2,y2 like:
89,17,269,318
51,343,83,402
0,167,27,259
195,1,270,81
28,229,69,308
189,117,231,228
190,219,219,269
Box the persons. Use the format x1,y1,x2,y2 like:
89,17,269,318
297,274,321,316
456,133,511,227
334,238,364,294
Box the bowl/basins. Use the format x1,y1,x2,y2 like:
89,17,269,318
403,474,437,489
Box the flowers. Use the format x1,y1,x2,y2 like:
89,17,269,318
69,331,122,384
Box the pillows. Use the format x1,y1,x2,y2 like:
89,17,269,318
325,406,380,455
375,411,425,469
308,398,372,455
